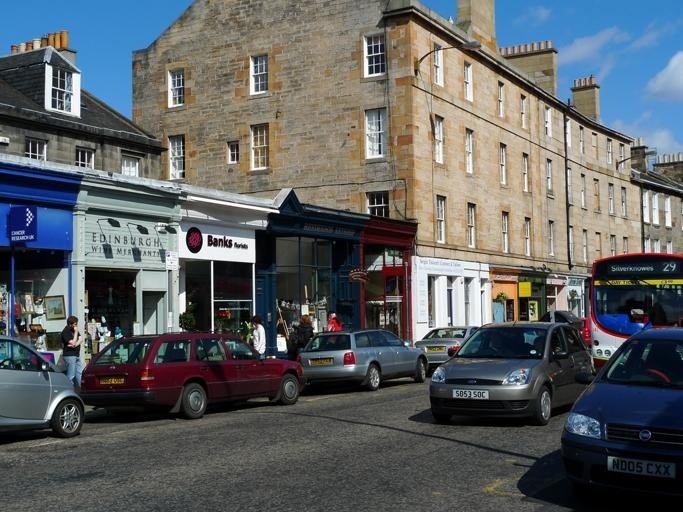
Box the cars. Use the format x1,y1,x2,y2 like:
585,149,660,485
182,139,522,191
414,325,480,377
560,327,682,499
0,335,85,437
429,320,595,425
537,309,578,324
579,317,591,347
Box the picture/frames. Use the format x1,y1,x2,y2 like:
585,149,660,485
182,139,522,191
44,295,66,320
19,292,36,315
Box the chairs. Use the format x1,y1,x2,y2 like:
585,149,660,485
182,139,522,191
163,348,188,362
645,348,683,376
534,336,552,353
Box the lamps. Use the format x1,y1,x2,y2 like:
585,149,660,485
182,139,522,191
414,40,482,76
567,289,577,302
155,224,177,233
128,222,148,234
96,218,121,229
615,148,657,170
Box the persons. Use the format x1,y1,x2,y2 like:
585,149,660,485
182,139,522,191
0,310,6,336
431,329,441,338
287,315,314,360
533,336,561,361
60,316,84,394
251,316,267,355
323,311,344,346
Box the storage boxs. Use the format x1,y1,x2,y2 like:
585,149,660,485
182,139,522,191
85,322,114,367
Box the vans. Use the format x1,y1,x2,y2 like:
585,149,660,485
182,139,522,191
79,332,303,419
296,328,428,391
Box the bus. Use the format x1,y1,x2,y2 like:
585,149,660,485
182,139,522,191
584,252,683,370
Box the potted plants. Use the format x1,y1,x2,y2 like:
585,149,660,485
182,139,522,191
493,291,508,323
